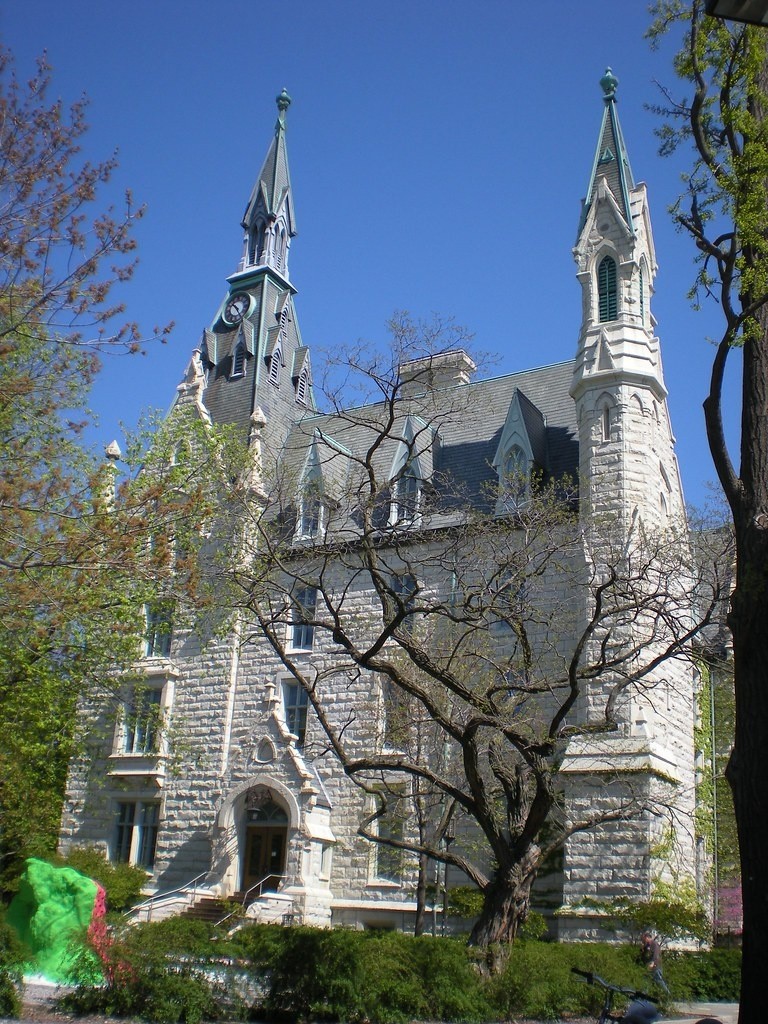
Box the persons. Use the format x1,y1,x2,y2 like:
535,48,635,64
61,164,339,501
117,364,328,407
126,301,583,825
640,932,672,996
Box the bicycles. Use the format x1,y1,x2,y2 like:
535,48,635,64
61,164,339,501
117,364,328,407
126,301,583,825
570,967,724,1024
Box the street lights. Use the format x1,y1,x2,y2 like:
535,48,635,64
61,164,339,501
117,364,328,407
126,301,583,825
702,643,729,949
